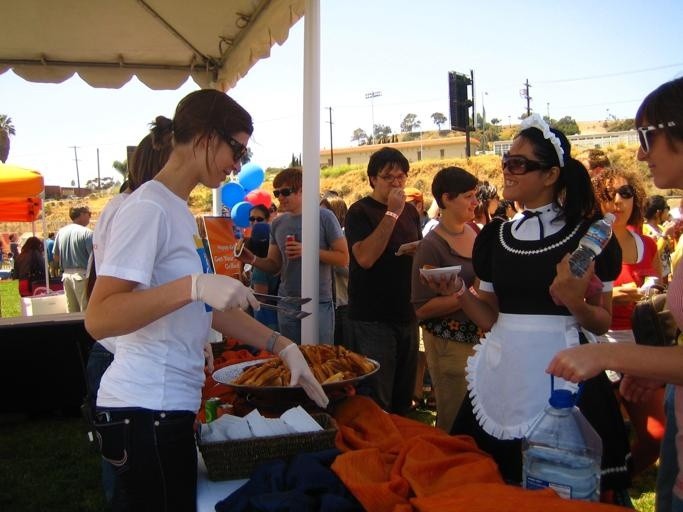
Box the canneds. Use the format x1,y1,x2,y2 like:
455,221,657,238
286,234,296,257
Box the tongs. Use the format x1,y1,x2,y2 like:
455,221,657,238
253,293,313,320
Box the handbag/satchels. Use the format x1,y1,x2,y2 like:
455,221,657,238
20,292,69,316
631,285,678,347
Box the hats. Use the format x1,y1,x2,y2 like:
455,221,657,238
403,187,423,203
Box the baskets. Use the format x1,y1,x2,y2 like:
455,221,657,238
196,413,338,481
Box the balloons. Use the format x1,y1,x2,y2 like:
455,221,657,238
221,182,246,210
231,201,254,228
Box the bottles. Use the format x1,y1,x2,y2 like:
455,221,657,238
568,212,616,277
64,270,83,314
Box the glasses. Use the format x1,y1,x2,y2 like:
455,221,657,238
273,188,296,198
637,121,679,154
81,211,91,216
249,216,265,222
600,185,634,202
377,174,407,182
216,127,247,160
665,206,670,210
502,151,548,175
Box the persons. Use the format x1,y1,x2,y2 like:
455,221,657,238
1,116,173,502
232,78,682,511
80,87,331,512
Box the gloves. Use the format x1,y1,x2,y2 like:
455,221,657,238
279,344,329,408
191,273,260,313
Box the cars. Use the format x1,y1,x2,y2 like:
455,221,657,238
66,194,80,199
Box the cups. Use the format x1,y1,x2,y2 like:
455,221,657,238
645,276,659,294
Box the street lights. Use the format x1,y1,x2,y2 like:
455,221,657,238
507,115,511,129
363,89,383,145
545,101,550,117
480,90,488,120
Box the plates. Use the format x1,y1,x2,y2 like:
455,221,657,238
421,264,462,284
213,356,379,398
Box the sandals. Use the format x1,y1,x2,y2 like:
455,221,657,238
408,385,436,411
